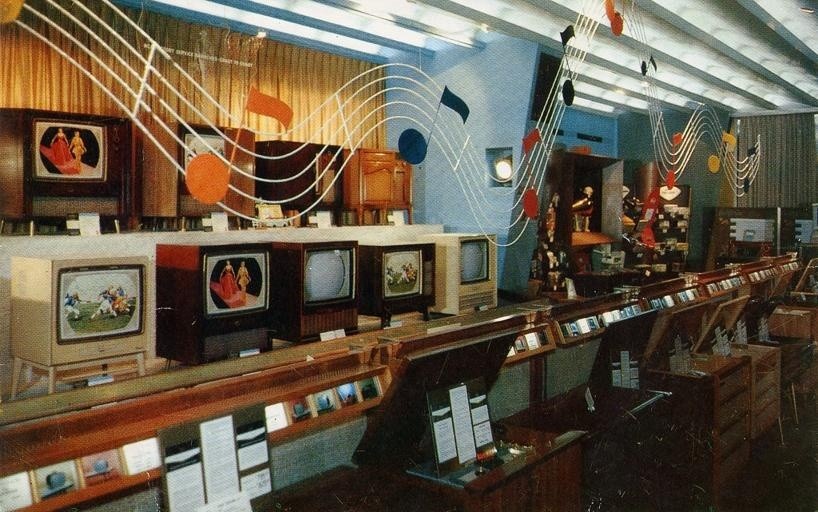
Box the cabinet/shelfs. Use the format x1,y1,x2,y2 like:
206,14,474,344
645,343,782,508
